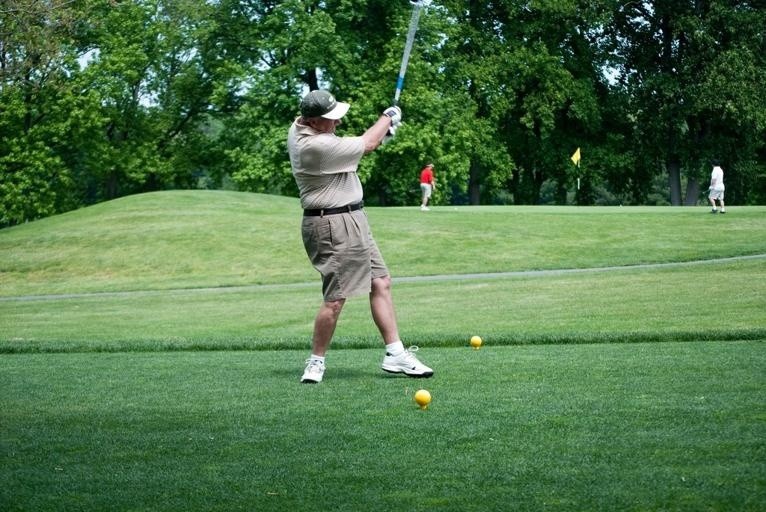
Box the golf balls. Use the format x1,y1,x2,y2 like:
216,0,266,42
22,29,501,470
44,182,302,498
471,336,482,347
414,389,431,407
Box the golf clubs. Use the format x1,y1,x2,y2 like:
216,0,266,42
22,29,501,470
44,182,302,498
393,0,432,105
702,189,709,194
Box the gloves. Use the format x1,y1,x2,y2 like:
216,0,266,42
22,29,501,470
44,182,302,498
384,105,403,136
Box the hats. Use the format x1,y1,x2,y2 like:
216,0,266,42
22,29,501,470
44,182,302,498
301,90,350,120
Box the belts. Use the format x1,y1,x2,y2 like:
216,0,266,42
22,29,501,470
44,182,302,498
303,199,364,217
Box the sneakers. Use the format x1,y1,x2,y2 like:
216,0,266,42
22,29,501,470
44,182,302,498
382,345,433,377
301,359,325,384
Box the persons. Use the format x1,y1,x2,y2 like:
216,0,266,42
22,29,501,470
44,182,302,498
708,159,726,213
420,163,435,211
288,89,433,382
